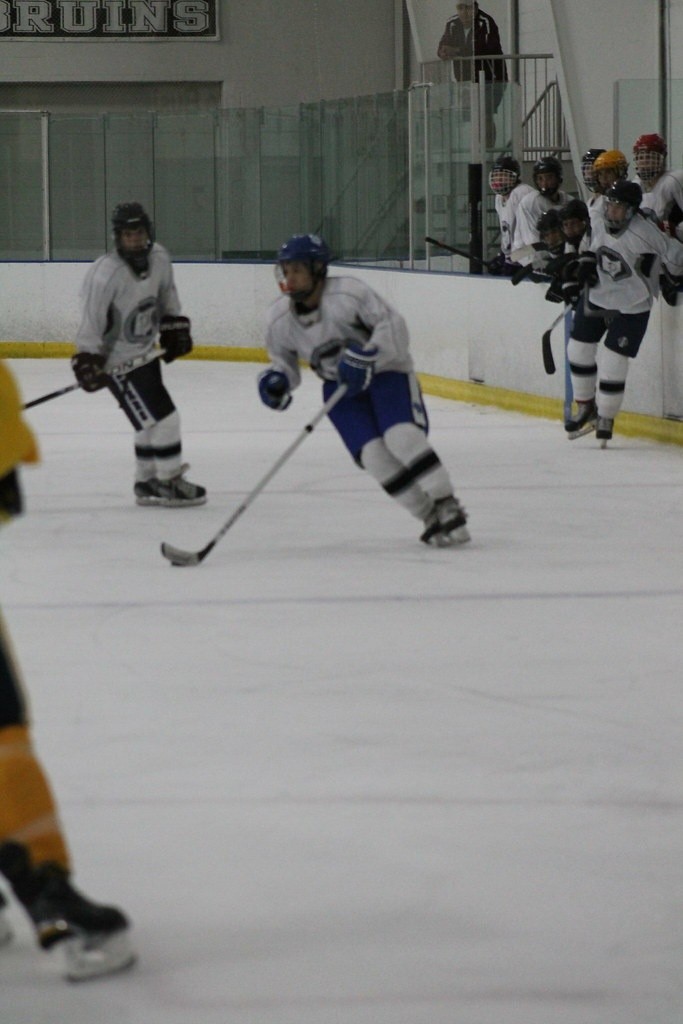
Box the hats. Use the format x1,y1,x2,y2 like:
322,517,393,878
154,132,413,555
276,234,329,314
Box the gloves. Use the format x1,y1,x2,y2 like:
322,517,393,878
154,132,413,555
158,314,193,364
335,339,379,395
533,248,597,307
257,370,293,411
71,352,108,393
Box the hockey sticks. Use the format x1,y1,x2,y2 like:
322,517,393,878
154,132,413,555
158,384,348,568
541,280,591,375
424,236,488,264
20,353,168,410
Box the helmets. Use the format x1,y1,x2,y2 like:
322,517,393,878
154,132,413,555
111,202,155,261
593,148,628,189
580,149,607,193
603,180,642,229
557,198,590,241
632,133,668,182
488,156,521,196
532,157,563,195
535,208,559,230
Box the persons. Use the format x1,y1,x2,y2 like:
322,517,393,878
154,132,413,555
487,132,683,441
0,356,136,955
436,0,509,159
68,200,208,500
254,232,468,543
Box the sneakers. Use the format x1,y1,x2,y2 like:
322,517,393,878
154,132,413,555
0,839,134,980
595,412,614,448
564,386,598,439
418,494,470,547
134,462,206,505
